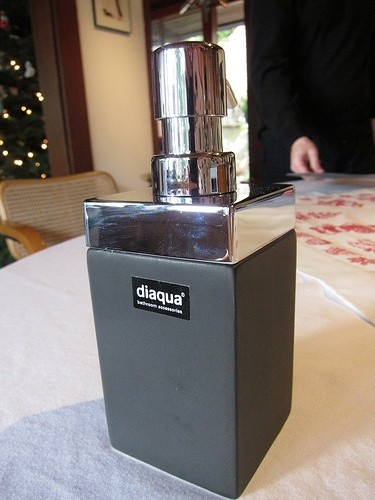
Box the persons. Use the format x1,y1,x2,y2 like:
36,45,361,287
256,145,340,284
275,0,375,173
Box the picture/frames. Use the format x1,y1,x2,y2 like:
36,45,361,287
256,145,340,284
92,0,132,34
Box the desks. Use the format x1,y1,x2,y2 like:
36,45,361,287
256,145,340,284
0,174,375,500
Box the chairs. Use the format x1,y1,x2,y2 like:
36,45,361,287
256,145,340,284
0,170,120,259
